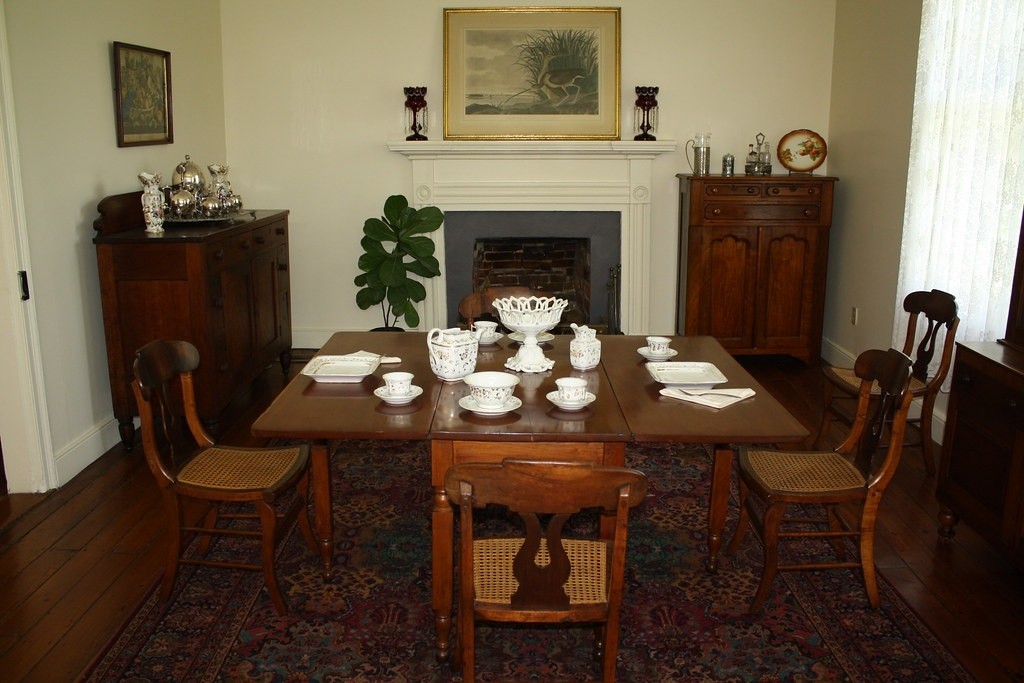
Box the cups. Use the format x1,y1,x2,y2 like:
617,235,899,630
646,336,672,355
569,323,601,370
382,372,414,396
555,377,588,403
474,321,498,340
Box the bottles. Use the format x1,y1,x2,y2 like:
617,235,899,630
746,142,771,165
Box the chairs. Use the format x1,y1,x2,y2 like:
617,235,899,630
443,457,648,683
730,347,913,625
131,338,324,618
459,285,555,333
814,290,961,495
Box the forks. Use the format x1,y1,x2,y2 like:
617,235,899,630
679,389,742,398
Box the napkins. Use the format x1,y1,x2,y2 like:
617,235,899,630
658,387,756,410
342,350,402,363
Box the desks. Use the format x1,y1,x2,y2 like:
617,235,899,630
91,182,293,464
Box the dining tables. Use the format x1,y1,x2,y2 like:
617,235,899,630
251,331,813,662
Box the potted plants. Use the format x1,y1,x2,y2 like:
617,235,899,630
354,195,446,332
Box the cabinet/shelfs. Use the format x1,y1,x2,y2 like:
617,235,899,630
676,171,840,368
935,339,1024,569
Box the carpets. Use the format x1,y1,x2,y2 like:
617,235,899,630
74,438,969,682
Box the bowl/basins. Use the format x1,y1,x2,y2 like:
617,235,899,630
463,371,521,409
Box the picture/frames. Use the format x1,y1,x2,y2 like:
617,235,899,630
442,8,622,141
113,40,174,148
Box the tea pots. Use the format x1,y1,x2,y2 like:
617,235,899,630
427,327,484,382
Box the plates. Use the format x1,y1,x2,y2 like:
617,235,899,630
637,346,678,362
644,362,728,389
373,385,423,405
459,395,522,416
546,390,596,410
777,129,827,172
479,332,504,344
300,354,381,383
507,333,555,345
